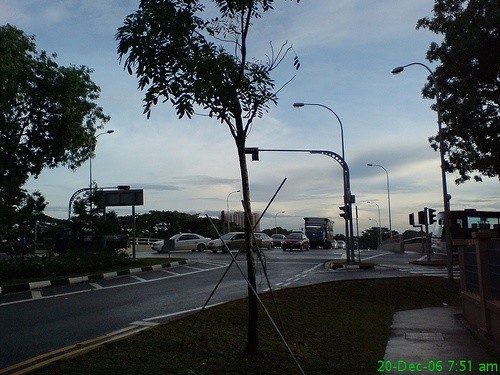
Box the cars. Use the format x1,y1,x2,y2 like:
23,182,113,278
281,232,310,251
207,232,263,253
270,234,286,248
332,239,346,249
253,232,274,250
150,232,212,253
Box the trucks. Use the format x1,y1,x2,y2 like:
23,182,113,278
301,217,335,250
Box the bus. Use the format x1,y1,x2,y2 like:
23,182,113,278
431,208,500,256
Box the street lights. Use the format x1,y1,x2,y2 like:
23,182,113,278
367,164,392,238
90,129,115,256
227,190,240,232
292,102,350,264
275,210,286,234
391,62,454,280
369,218,379,246
367,202,382,244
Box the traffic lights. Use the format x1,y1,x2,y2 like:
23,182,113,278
428,209,438,224
339,204,350,220
